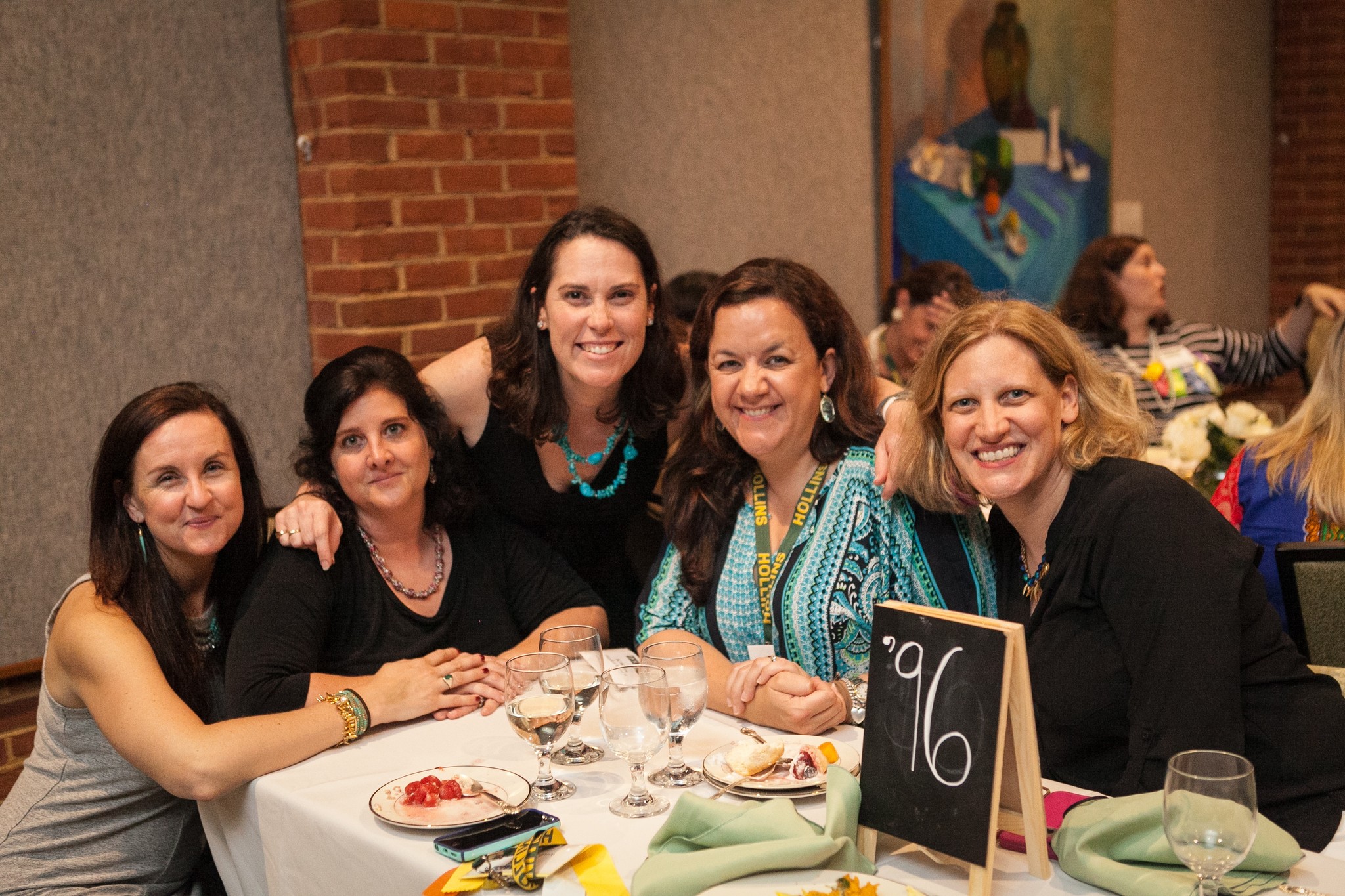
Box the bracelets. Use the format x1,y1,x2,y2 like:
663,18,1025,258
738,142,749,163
315,687,373,749
838,675,866,726
874,389,916,424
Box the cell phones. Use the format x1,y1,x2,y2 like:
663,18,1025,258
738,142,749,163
432,807,561,863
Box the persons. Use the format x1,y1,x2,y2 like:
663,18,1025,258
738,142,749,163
658,273,717,355
853,259,987,387
1051,235,1344,487
272,204,920,648
633,257,999,736
875,297,1345,868
221,350,614,722
1199,309,1344,656
0,380,489,896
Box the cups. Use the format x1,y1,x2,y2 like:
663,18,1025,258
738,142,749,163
1251,402,1286,435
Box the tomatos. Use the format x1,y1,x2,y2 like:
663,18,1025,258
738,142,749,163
401,775,461,806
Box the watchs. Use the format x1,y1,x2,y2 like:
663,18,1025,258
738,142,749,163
848,676,868,708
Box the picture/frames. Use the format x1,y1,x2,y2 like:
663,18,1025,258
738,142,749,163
869,0,1121,333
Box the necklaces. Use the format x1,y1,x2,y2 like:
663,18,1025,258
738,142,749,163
549,403,638,499
187,601,221,658
1016,530,1050,602
355,518,447,600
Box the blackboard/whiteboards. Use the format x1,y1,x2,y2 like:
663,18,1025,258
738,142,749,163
856,599,1049,869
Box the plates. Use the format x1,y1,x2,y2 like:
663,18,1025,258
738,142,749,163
369,765,532,829
702,734,861,799
694,869,928,896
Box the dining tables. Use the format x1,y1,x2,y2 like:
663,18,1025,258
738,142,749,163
189,647,1344,896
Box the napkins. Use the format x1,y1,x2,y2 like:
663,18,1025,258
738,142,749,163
1048,787,1308,896
629,763,880,896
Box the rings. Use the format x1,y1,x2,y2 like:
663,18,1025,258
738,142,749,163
275,528,287,539
478,695,486,708
442,675,453,691
768,655,776,663
287,529,300,536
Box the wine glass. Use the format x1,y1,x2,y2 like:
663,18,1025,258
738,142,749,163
639,642,707,788
1162,749,1258,896
536,625,605,765
599,663,671,819
505,652,576,802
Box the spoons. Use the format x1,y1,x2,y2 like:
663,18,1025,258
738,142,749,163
707,763,776,800
451,772,521,814
740,727,793,767
